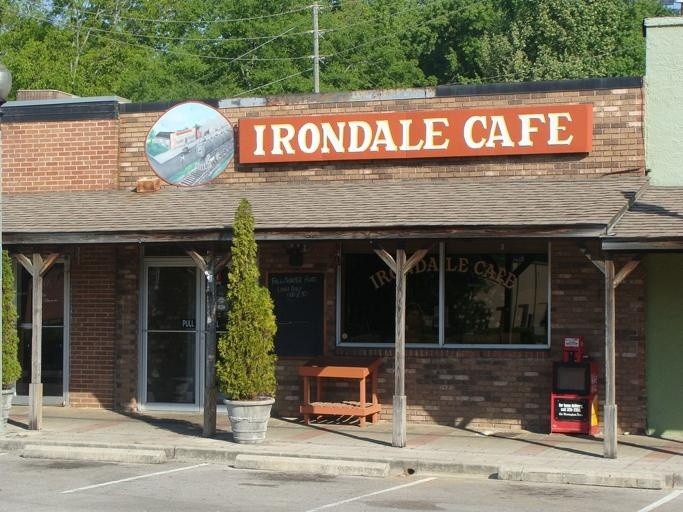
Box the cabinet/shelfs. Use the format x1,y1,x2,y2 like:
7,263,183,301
297,354,380,426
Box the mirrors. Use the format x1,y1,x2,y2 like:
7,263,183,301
144,100,236,187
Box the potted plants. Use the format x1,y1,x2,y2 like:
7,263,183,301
216,198,280,443
0,250,23,423
